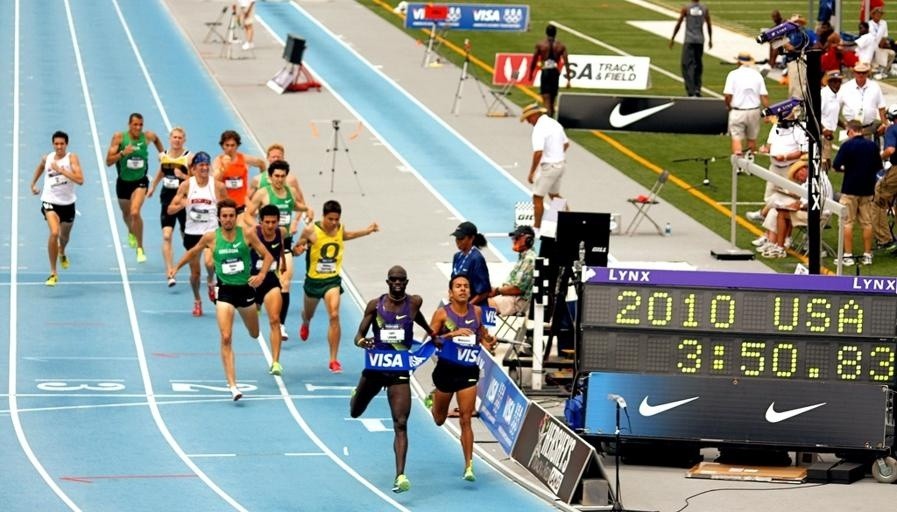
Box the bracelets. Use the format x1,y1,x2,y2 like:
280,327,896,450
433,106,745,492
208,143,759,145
120,150,125,159
428,329,436,336
495,287,500,296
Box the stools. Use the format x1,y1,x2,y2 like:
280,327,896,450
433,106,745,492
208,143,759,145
790,224,837,256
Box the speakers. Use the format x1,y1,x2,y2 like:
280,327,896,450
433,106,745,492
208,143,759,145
282,34,305,64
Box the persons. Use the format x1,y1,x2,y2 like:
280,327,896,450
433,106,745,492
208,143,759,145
349,266,443,489
105,114,164,263
213,131,265,216
724,53,768,175
31,132,84,289
145,128,196,286
234,0,256,50
764,9,896,159
249,143,304,239
424,274,497,482
246,205,287,375
487,224,537,317
167,199,273,401
292,200,378,374
528,24,571,118
243,160,312,340
167,152,233,316
519,103,569,234
445,221,490,417
746,107,833,260
834,120,882,266
669,0,712,97
870,106,896,250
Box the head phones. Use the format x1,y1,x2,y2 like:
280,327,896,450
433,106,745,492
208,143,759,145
886,107,894,121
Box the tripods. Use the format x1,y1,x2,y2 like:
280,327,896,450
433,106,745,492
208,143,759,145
219,13,257,62
312,119,364,199
451,48,490,118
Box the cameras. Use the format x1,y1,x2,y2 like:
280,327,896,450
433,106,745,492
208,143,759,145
233,5,236,13
464,38,470,48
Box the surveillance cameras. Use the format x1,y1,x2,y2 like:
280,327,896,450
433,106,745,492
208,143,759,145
756,23,799,44
761,98,800,118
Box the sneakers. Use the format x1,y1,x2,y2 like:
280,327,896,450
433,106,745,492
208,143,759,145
391,473,411,494
130,231,341,402
46,273,59,286
59,254,70,269
462,465,476,482
745,209,894,268
423,388,437,409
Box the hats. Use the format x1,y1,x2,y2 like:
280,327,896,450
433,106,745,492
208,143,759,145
508,226,534,237
520,103,549,122
733,52,897,180
449,222,477,238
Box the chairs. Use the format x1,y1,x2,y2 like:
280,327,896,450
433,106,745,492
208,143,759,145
625,170,669,236
204,7,227,44
485,72,519,118
493,298,529,340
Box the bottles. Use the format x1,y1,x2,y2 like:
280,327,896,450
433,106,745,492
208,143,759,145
664,222,672,237
